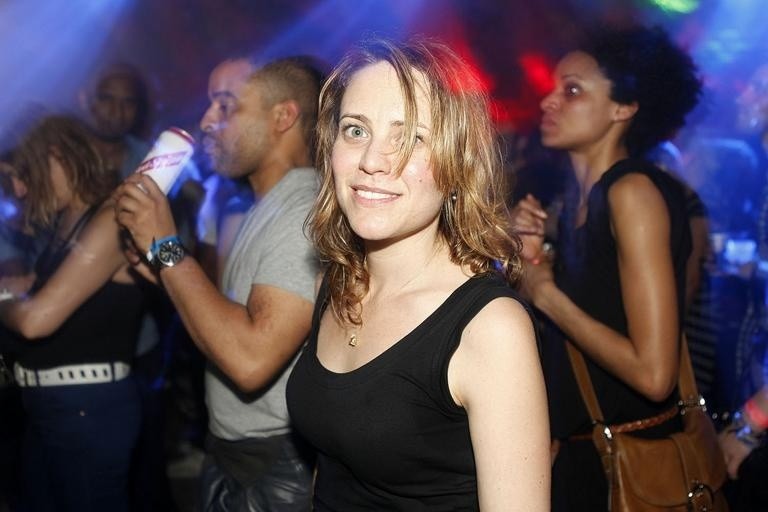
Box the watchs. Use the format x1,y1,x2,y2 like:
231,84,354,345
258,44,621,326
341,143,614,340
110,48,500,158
149,240,191,280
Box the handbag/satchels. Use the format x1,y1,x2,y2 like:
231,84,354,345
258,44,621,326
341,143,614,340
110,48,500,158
594,406,738,512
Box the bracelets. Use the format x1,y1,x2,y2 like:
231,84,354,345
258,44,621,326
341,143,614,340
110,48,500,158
531,243,552,265
0,290,15,302
142,235,179,265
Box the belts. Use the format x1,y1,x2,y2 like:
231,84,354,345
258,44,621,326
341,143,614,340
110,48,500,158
13,357,132,387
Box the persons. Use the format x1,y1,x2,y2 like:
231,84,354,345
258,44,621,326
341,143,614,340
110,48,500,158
0,101,147,511
179,158,255,296
640,63,768,512
510,20,704,512
116,50,329,511
284,30,552,512
77,72,152,183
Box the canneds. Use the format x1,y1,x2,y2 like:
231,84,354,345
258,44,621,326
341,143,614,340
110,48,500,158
133,126,196,199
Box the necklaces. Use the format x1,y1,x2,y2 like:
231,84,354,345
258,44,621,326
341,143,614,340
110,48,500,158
348,256,440,348
